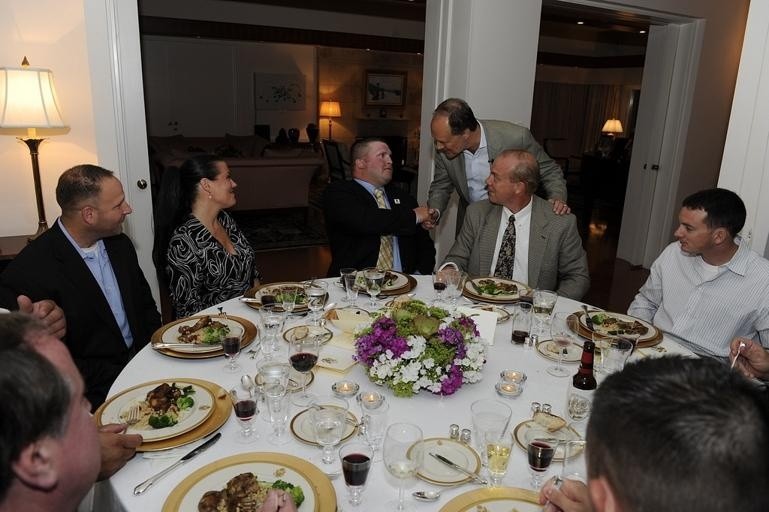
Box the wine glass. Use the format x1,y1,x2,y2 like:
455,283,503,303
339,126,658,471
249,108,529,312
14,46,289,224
427,268,469,314
257,281,296,358
534,290,557,337
308,397,349,476
230,384,261,444
343,268,387,310
289,335,320,405
381,421,424,512
359,397,390,463
220,328,246,372
302,280,326,325
256,357,294,444
548,312,580,377
337,443,376,509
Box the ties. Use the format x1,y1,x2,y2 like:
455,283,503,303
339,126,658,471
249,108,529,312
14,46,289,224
494,215,517,281
373,189,393,271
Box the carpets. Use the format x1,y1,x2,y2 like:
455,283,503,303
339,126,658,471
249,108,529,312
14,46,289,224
240,214,330,254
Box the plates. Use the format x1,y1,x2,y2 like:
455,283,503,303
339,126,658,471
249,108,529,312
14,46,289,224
91,377,238,455
537,340,584,363
245,283,328,316
408,437,482,484
346,271,417,297
470,304,509,324
579,310,643,340
569,308,663,351
160,451,340,511
149,311,258,360
303,345,362,370
283,324,332,345
513,418,585,463
465,277,530,305
438,486,559,511
291,405,357,444
254,364,312,394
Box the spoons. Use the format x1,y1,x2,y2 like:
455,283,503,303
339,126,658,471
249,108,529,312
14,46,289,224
411,486,451,502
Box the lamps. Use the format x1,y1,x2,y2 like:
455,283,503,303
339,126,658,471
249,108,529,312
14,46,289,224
319,97,342,141
0,56,71,244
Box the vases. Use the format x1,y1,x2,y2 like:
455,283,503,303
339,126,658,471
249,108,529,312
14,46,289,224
288,128,300,142
306,123,319,143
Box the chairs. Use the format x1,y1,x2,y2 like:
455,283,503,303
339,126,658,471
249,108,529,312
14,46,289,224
322,140,352,182
390,135,415,196
542,137,584,198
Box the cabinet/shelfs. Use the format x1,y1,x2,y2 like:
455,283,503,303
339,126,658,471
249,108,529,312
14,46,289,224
580,150,630,203
1,236,34,305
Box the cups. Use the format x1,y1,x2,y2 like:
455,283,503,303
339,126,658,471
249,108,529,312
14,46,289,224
482,429,516,481
470,398,511,469
524,429,560,483
510,303,534,347
517,286,533,310
588,326,634,374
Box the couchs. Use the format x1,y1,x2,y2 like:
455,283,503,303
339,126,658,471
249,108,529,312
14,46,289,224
151,134,327,211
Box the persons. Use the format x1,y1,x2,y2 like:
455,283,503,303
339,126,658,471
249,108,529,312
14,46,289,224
95,421,141,482
0,164,160,414
421,97,572,240
537,350,768,511
0,293,104,508
17,292,67,341
163,151,262,321
623,187,769,366
429,148,590,301
724,332,768,385
319,134,436,277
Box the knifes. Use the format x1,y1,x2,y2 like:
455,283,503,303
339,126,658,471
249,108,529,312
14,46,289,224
134,433,224,497
434,452,487,484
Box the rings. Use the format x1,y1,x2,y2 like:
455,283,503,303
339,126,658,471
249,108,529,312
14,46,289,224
563,204,569,209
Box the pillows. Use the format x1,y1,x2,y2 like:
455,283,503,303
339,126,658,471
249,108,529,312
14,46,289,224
225,133,271,159
150,135,206,150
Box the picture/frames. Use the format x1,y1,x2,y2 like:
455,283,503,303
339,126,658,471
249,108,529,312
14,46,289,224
361,68,408,110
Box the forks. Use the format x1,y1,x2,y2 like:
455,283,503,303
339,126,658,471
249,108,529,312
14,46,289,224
580,304,595,331
217,305,227,323
121,400,140,440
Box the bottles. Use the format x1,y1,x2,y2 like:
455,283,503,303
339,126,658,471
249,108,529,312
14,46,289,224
542,402,552,414
528,402,542,417
564,338,598,432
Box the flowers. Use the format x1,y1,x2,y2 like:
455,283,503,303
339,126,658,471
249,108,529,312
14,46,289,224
351,299,475,402
